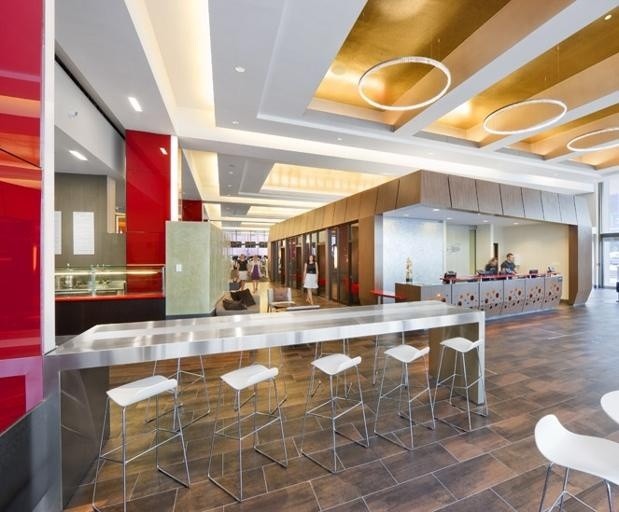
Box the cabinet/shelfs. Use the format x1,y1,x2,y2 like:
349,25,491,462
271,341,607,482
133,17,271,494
53,262,164,299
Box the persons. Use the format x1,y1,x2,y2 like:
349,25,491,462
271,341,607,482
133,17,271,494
237,254,250,290
232,254,270,280
303,254,320,306
249,255,263,293
501,253,518,275
485,258,498,271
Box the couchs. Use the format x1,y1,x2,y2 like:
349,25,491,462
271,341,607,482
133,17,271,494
219,291,260,314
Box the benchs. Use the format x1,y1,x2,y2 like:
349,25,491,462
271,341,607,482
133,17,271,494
369,288,408,304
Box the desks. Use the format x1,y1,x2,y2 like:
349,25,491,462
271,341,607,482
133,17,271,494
43,299,488,511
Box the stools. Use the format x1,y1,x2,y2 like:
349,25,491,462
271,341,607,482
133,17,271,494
91,374,190,512
600,390,619,427
534,413,619,512
300,352,371,474
206,364,291,503
373,344,435,450
431,336,489,432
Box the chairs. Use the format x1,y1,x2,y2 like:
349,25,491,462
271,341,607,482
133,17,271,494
267,287,294,312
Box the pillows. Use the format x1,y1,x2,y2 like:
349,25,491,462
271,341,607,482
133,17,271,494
223,297,246,311
229,288,255,305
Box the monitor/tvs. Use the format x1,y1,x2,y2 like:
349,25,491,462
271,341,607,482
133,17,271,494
443,272,456,284
528,269,538,278
